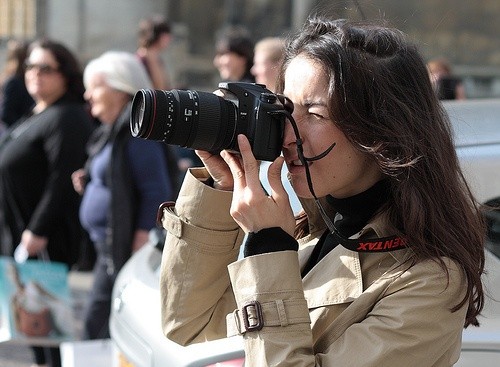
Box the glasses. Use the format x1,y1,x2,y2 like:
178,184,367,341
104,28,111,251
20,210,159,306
24,63,60,73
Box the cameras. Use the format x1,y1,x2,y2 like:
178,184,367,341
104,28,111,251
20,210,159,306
130,82,294,161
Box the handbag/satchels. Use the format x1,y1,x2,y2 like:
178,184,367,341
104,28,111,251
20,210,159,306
0,247,85,348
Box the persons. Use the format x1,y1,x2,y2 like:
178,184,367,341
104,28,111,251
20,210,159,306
71,50,171,339
0,39,88,367
159,10,489,367
213,22,464,101
139,22,172,91
